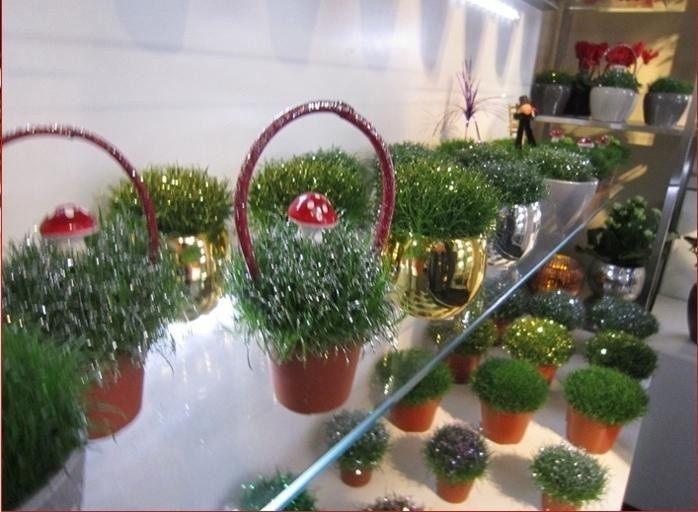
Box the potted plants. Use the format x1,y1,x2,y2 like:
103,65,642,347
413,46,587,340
474,159,545,269
394,161,497,320
389,143,438,161
643,78,693,126
439,139,507,167
577,197,662,299
528,146,598,234
109,170,231,320
239,471,314,512
230,219,388,412
426,307,496,384
684,236,698,342
2,332,89,511
330,409,388,486
504,316,574,385
551,129,622,190
531,71,572,117
564,366,649,452
589,71,638,122
480,275,530,324
474,360,546,444
534,446,607,511
587,330,658,381
496,140,532,159
427,425,489,504
250,149,376,243
589,297,660,338
570,41,660,116
530,290,584,330
364,494,423,511
1,219,181,440
379,350,451,432
